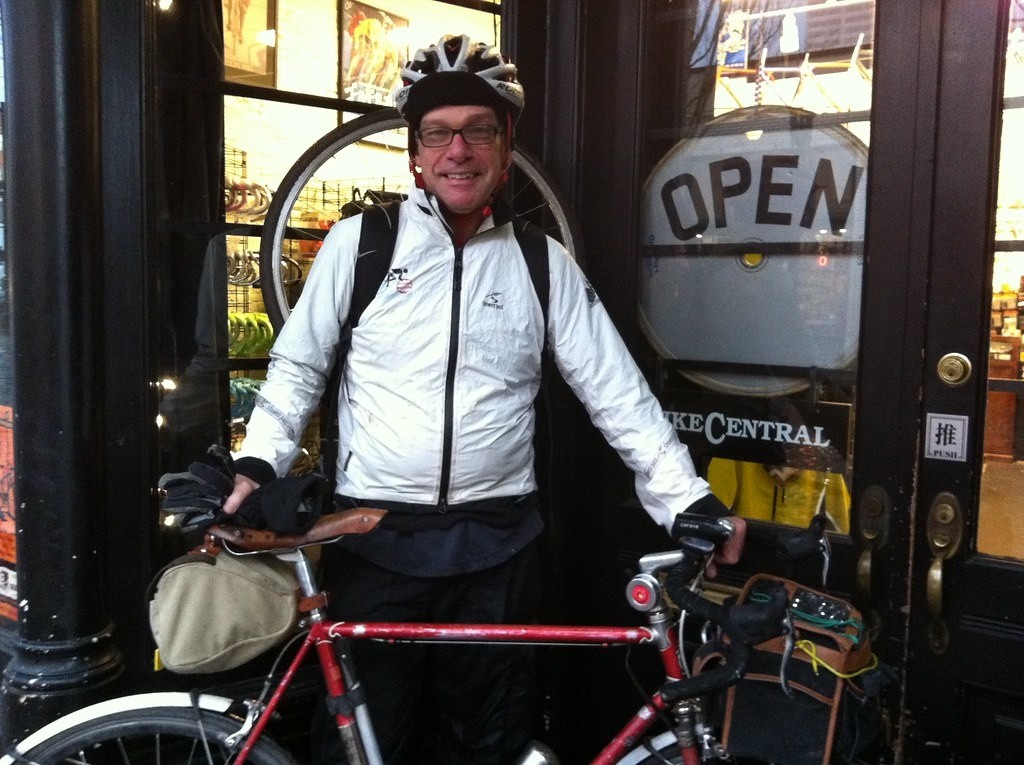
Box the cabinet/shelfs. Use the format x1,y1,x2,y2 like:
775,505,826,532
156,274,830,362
226,222,340,479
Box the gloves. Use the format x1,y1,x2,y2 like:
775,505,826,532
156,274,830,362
157,445,329,537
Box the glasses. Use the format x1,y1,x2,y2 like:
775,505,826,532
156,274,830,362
415,125,505,149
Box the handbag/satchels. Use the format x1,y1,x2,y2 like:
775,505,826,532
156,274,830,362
148,534,299,674
691,572,877,764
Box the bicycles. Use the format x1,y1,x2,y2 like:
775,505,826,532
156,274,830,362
0,508,892,765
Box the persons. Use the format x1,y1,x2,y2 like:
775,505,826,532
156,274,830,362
222,35,746,765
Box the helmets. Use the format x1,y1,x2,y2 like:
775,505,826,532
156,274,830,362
396,34,524,124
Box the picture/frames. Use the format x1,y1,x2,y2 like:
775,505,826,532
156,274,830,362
222,0,279,92
336,0,410,150
